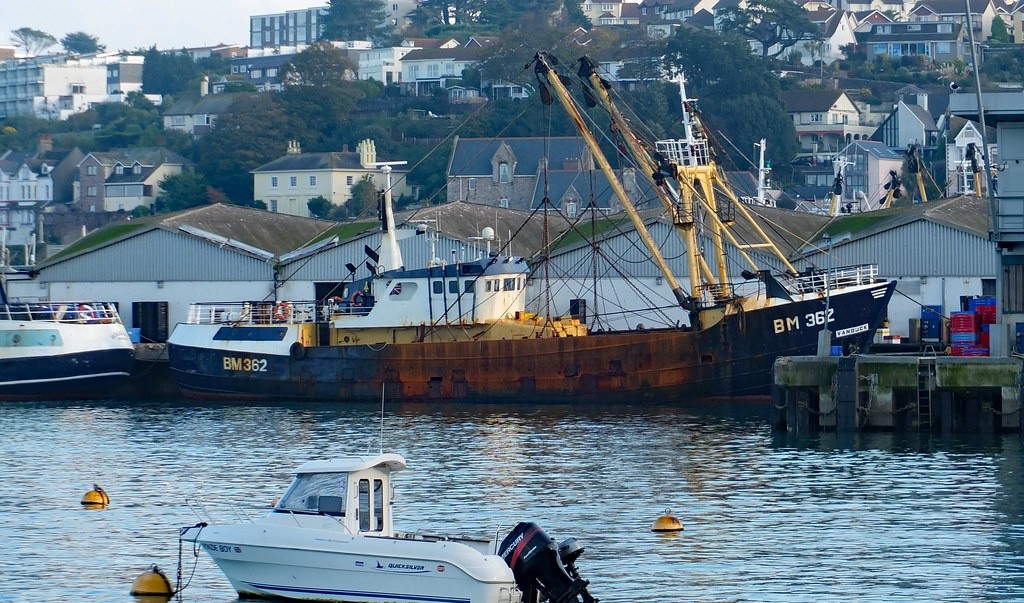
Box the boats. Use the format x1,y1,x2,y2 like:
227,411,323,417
0,277,137,402
165,47,898,401
178,451,601,603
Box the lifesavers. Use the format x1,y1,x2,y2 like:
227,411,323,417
328,296,342,309
275,302,290,319
351,291,364,307
79,304,95,320
